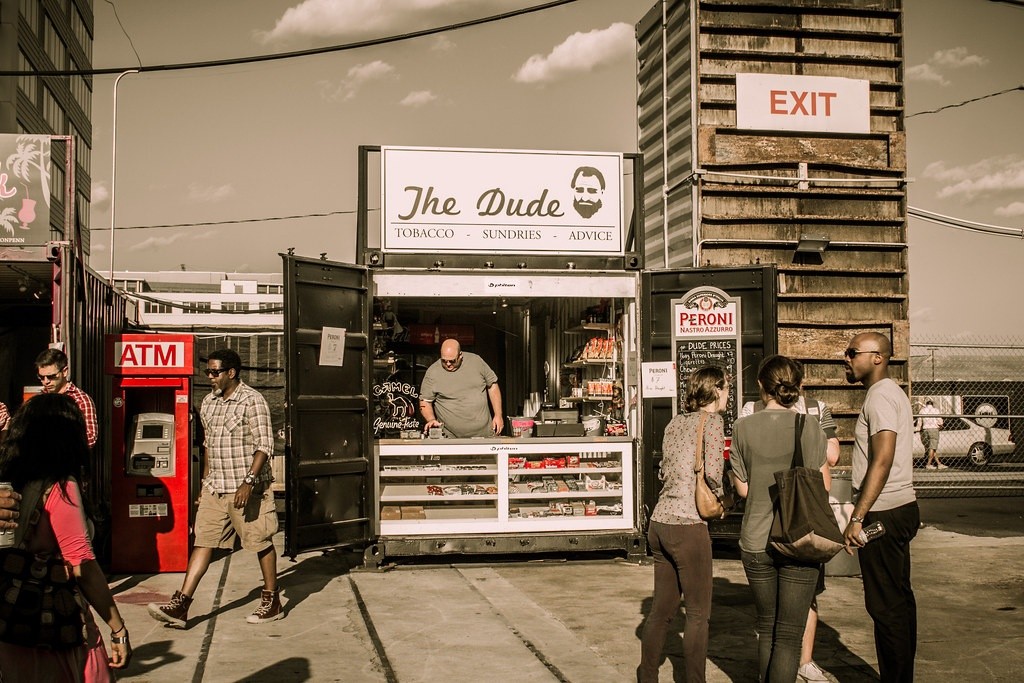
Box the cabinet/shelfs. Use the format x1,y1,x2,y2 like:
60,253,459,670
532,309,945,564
373,439,636,535
562,319,617,403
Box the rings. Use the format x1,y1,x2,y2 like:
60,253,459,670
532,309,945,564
242,502,244,503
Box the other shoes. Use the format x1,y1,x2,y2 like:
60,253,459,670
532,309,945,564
925,464,937,470
938,464,948,469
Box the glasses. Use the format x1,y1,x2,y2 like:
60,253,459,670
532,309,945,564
441,351,462,363
845,349,884,360
38,365,67,380
203,367,236,377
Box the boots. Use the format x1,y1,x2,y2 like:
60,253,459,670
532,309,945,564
246,585,284,624
148,590,194,629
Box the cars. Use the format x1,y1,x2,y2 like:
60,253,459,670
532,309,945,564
912,414,1016,465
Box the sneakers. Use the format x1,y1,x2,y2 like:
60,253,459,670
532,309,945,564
795,662,830,683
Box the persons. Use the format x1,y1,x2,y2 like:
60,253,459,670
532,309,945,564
636,366,728,683
842,332,921,683
729,353,840,683
147,348,285,624
1,348,132,683
915,401,948,469
419,338,504,437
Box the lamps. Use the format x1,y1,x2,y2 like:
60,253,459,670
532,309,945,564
792,234,830,264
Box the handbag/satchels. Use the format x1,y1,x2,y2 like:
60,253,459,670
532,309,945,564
768,411,847,563
0,475,87,652
694,412,727,519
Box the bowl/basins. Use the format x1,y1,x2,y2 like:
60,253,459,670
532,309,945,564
507,415,537,437
580,415,606,436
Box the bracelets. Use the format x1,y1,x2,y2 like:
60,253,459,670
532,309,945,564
110,630,129,644
850,517,864,523
112,617,125,634
247,471,259,483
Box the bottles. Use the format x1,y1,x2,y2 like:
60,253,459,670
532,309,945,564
850,521,886,549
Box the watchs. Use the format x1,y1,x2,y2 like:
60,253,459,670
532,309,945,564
243,476,256,486
